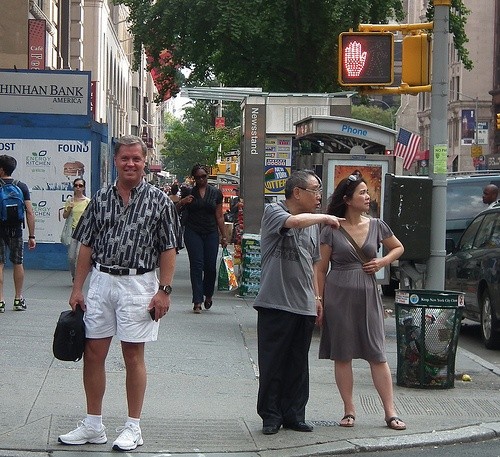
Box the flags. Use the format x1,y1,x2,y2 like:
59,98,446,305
395,128,420,170
149,51,183,100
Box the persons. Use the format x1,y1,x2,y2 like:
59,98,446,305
63,179,92,282
162,182,193,211
175,165,228,313
318,174,408,430
50,135,184,450
0,155,35,312
252,171,346,435
482,183,499,209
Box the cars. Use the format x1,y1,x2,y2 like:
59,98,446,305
444,197,500,350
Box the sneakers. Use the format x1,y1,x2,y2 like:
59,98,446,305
57,418,107,445
0,300,6,313
13,295,28,311
112,421,143,451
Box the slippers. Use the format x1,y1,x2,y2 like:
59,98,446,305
385,416,406,430
340,414,355,427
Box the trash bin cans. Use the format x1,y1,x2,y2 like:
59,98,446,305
394,287,466,389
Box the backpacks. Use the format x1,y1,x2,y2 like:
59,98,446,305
0,178,27,229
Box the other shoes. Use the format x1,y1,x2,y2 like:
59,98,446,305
262,421,282,435
193,304,202,314
204,296,212,309
284,421,314,432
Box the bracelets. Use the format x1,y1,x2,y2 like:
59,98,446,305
28,235,34,239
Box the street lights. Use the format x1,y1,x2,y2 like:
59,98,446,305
370,98,396,131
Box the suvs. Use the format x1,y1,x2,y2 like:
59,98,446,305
446,168,500,245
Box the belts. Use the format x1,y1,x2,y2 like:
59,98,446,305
93,261,155,275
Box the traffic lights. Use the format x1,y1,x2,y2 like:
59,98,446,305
496,113,500,130
337,32,395,87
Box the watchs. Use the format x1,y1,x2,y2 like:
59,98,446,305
316,296,323,301
159,284,172,295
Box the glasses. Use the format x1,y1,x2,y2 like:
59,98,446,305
74,184,84,188
194,174,207,179
291,186,323,194
347,175,359,184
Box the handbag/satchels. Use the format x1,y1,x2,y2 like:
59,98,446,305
218,244,238,293
174,205,185,252
61,209,72,245
53,304,86,362
